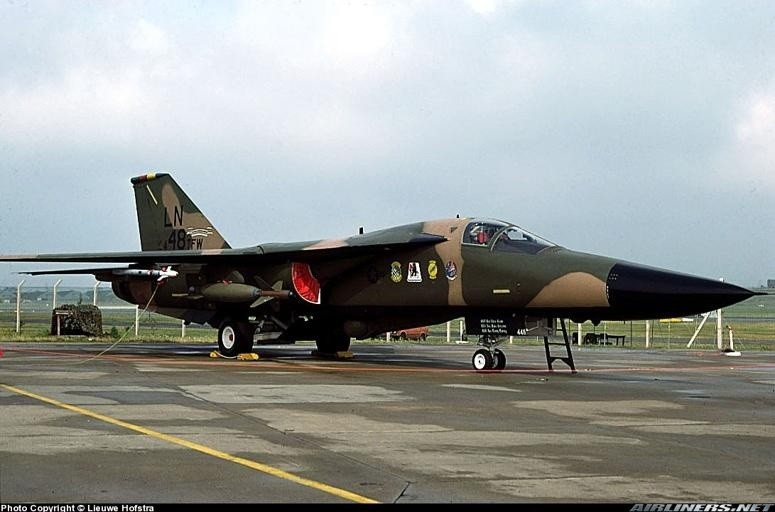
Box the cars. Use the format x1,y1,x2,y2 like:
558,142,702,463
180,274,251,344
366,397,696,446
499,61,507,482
382,326,431,341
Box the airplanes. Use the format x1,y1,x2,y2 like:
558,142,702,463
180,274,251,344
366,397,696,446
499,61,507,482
0,166,774,374
